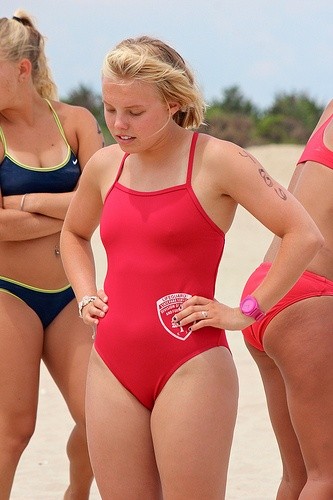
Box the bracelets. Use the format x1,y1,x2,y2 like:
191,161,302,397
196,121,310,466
19,191,32,213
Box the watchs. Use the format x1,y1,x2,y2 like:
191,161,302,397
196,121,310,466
78,295,98,318
238,293,268,325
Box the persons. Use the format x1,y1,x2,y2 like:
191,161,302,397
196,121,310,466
58,35,327,500
0,8,108,500
238,94,333,500
